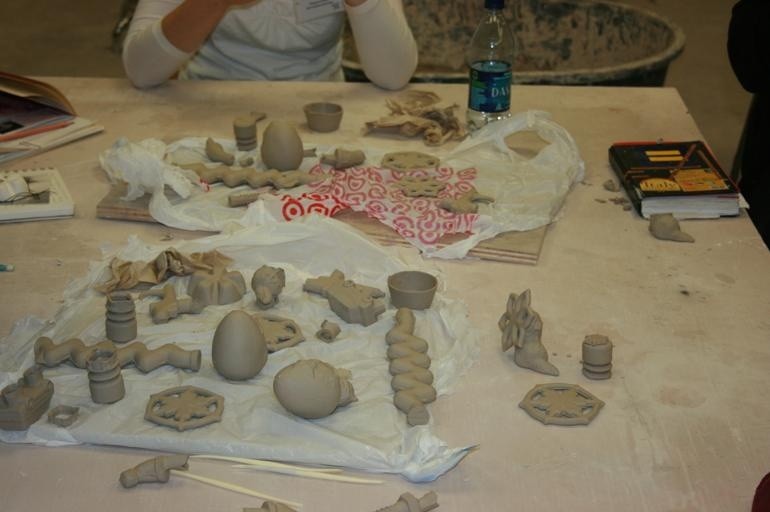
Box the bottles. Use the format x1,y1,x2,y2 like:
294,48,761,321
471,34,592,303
466,0,513,136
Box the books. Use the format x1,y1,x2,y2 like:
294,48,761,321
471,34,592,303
609,140,749,221
0,165,74,224
1,71,103,163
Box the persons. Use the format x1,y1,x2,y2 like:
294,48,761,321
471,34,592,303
121,1,419,91
727,1,769,253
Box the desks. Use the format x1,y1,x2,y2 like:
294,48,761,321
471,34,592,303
0,74,769,512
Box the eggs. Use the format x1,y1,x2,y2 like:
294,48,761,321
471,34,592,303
274,361,341,418
211,309,268,381
261,119,302,172
